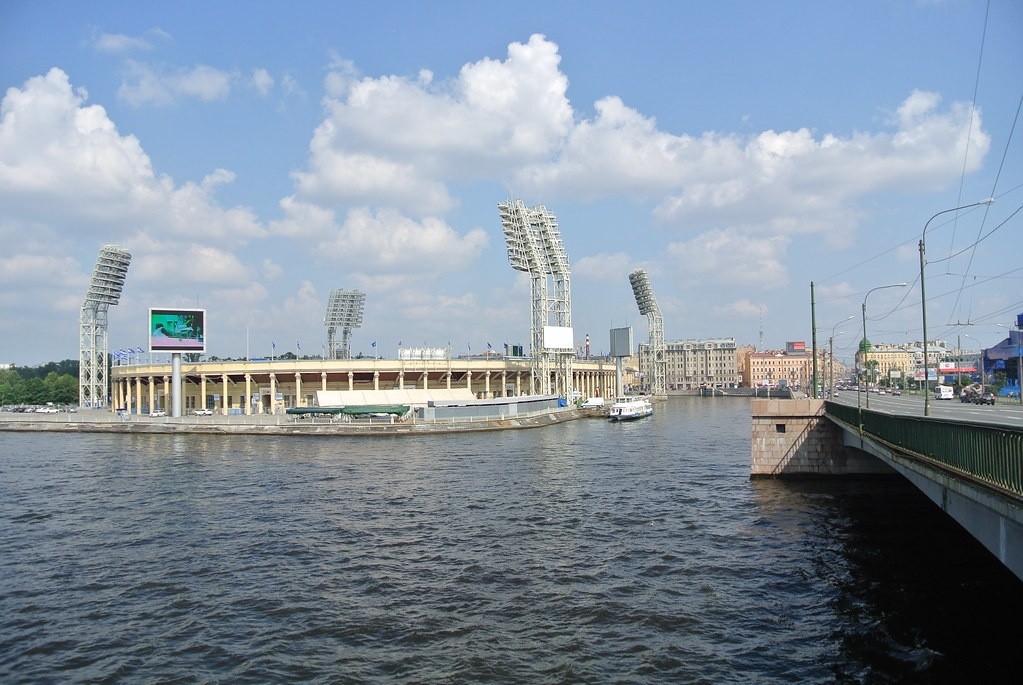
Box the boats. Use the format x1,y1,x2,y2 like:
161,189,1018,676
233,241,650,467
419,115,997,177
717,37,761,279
609,395,653,421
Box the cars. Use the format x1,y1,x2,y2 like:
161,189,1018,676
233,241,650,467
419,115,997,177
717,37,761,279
868,387,873,392
975,392,995,406
3,406,77,414
835,385,858,391
892,390,901,396
860,387,866,392
885,388,892,393
149,410,166,417
194,409,212,416
959,389,979,404
833,390,839,397
878,390,885,395
873,387,879,393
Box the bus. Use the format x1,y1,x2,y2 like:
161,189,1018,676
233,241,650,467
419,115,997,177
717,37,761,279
934,385,954,400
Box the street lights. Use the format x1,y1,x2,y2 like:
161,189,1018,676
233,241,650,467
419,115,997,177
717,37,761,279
918,198,995,419
997,324,1023,405
830,316,856,400
964,335,984,394
823,332,844,400
862,282,907,409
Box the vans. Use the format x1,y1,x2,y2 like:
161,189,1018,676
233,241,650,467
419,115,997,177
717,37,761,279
581,398,605,408
